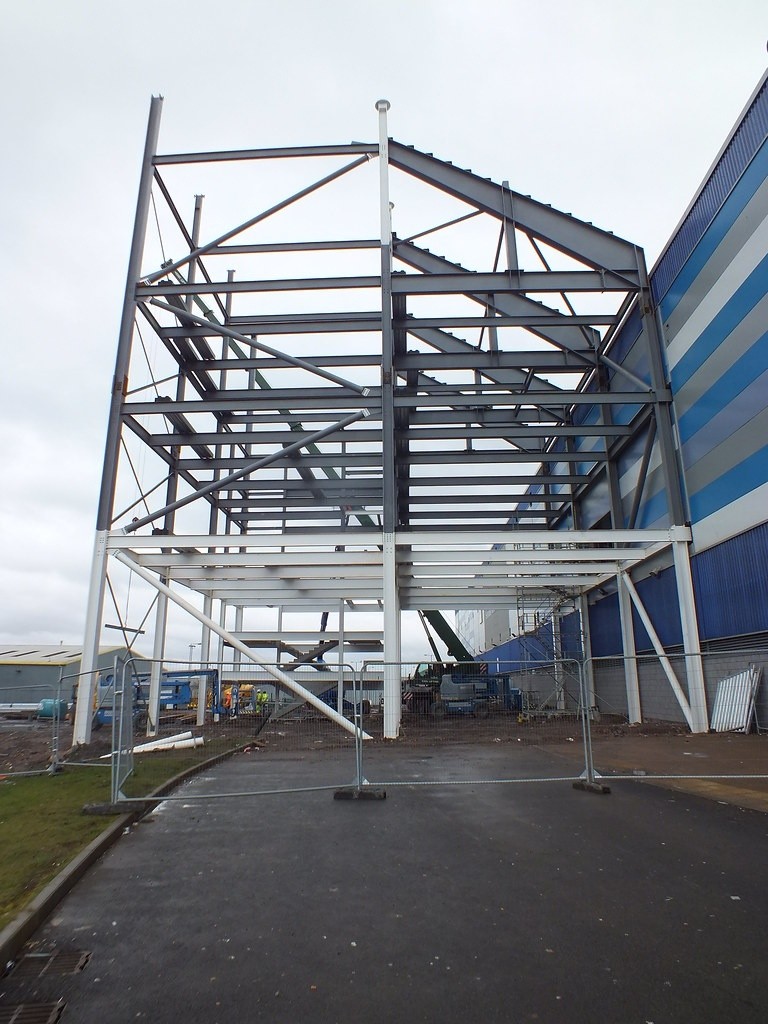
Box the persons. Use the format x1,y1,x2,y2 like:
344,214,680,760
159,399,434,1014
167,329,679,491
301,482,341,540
256,689,262,713
261,691,268,708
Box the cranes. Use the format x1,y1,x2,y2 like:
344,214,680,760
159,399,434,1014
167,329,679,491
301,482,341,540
155,259,492,718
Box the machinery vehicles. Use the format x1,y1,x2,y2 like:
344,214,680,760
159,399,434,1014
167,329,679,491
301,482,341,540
430,673,523,718
89,669,221,729
